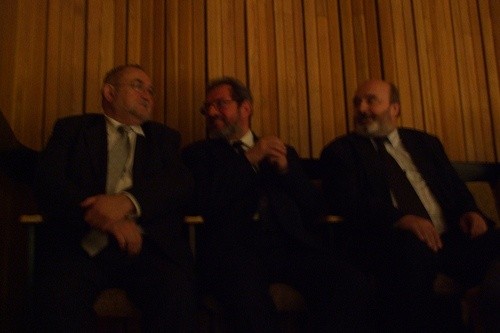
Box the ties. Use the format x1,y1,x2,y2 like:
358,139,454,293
372,136,434,224
233,140,261,212
106,124,131,193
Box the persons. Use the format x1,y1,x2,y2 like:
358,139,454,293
317,78,500,333
172,74,327,333
34,64,218,333
1,114,52,333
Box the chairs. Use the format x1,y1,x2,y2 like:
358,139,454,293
0,112,500,333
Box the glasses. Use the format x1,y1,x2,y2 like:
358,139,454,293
199,99,232,116
